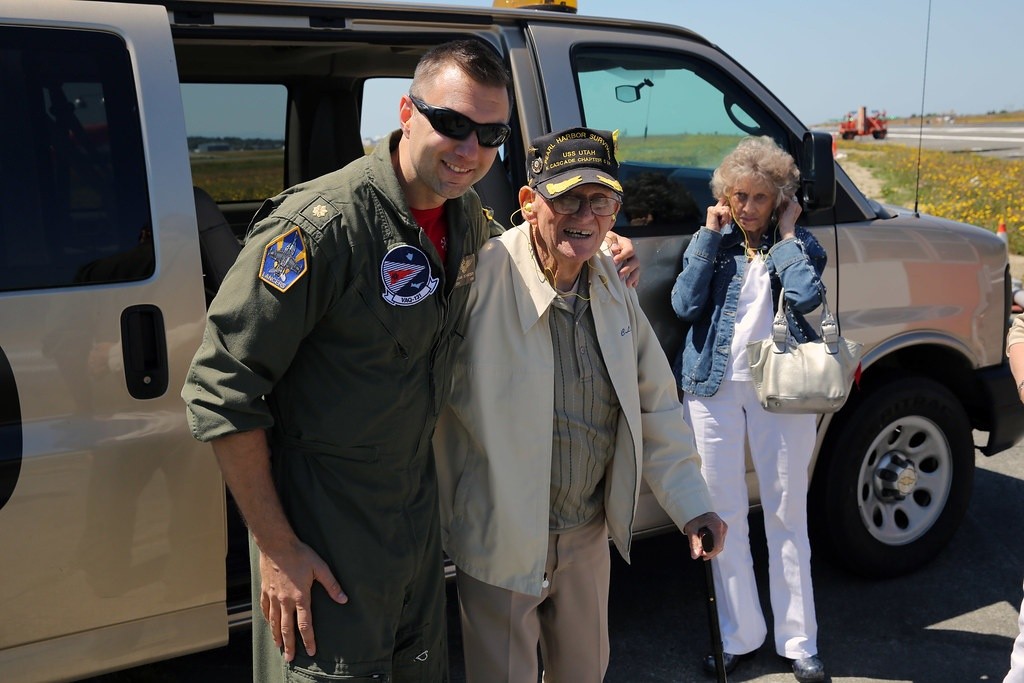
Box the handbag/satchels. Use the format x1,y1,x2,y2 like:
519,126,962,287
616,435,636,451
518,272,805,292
744,286,863,414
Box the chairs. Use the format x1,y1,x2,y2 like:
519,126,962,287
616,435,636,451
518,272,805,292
194,185,241,291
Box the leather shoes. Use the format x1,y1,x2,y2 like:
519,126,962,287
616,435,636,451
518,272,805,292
790,655,827,682
704,651,755,677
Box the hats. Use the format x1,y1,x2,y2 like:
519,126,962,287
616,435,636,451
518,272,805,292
526,126,624,199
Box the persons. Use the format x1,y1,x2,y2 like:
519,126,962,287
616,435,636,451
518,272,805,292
670,137,831,683
431,128,727,683
182,39,640,683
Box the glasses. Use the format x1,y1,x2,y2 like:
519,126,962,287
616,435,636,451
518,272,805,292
535,188,623,216
409,94,511,147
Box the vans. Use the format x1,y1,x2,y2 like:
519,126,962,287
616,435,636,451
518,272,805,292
0,0,1024,683
